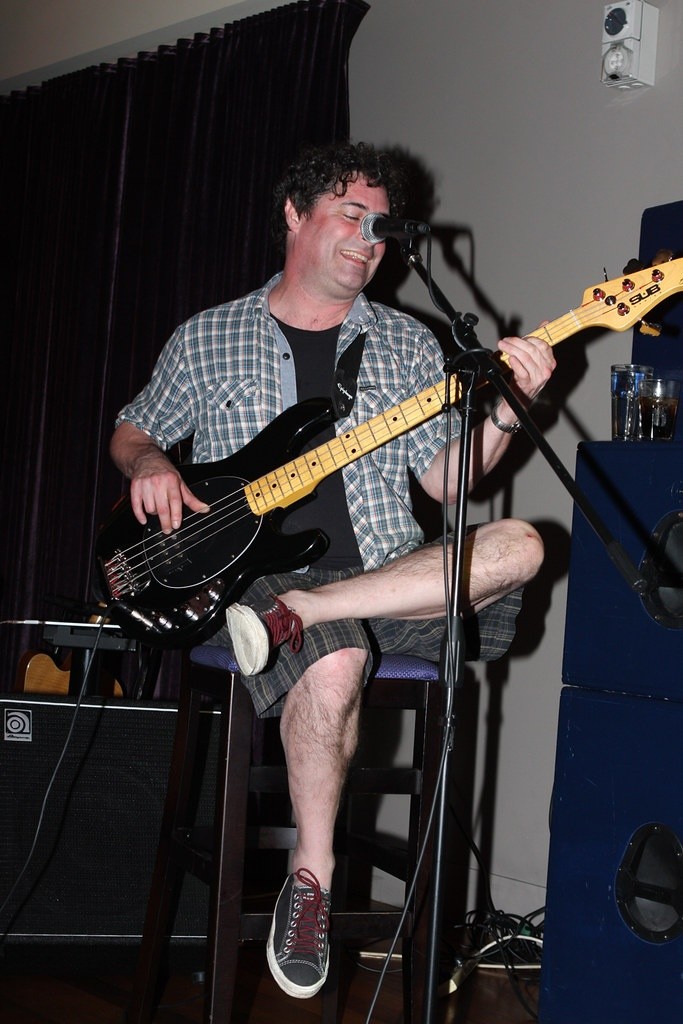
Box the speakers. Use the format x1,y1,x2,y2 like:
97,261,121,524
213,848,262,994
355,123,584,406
0,692,223,945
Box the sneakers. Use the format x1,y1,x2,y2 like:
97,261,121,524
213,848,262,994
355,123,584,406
226,593,305,678
267,868,333,1001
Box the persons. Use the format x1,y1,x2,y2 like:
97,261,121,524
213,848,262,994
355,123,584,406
106,145,561,999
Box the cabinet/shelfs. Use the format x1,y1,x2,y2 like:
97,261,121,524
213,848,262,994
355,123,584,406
536,439,683,1024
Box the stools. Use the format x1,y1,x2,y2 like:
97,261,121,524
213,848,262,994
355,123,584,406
127,646,440,1024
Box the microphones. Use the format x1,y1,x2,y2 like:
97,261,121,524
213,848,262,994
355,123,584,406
360,212,431,243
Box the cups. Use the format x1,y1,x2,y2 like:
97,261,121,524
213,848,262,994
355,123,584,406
639,379,679,441
611,364,654,441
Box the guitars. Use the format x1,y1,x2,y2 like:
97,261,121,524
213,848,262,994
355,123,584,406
91,238,683,651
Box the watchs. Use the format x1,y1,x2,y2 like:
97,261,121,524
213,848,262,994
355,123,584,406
489,407,521,436
